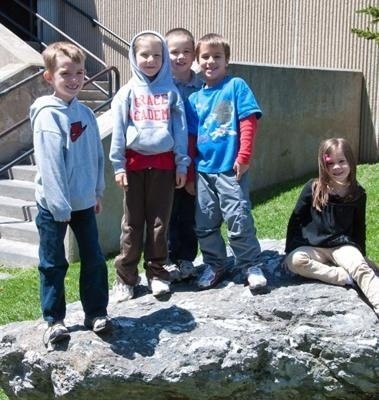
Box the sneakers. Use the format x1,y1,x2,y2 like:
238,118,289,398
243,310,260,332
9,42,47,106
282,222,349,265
48,320,69,343
148,276,171,297
114,275,141,301
197,265,226,288
242,263,268,290
84,313,112,332
162,258,196,282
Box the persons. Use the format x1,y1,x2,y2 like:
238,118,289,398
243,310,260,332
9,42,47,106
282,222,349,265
107,29,192,303
280,136,379,318
182,32,268,292
161,27,204,285
30,40,111,345
